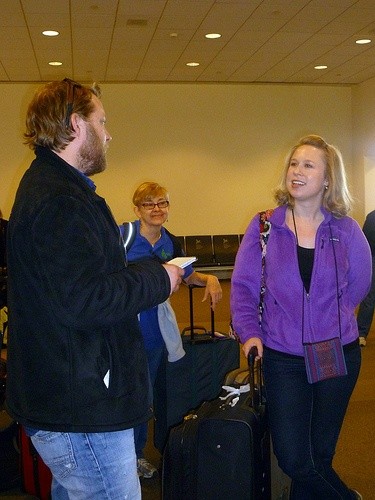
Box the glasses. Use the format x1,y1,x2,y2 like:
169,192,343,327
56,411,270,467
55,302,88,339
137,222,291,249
138,201,169,210
63,77,82,129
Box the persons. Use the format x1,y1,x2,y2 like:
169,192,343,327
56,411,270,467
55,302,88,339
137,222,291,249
230,135,375,500
119,181,223,479
4,76,184,500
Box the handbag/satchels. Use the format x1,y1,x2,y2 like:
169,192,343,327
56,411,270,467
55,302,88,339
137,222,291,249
304,338,348,383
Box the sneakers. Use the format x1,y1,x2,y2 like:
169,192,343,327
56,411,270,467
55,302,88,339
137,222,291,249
136,459,157,479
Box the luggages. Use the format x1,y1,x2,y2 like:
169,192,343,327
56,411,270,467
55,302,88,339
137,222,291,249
153,284,239,456
161,348,271,500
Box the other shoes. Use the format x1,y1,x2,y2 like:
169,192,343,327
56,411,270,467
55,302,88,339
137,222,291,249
359,337,368,346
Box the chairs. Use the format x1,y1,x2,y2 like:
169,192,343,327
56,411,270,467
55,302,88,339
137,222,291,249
171,233,246,282
